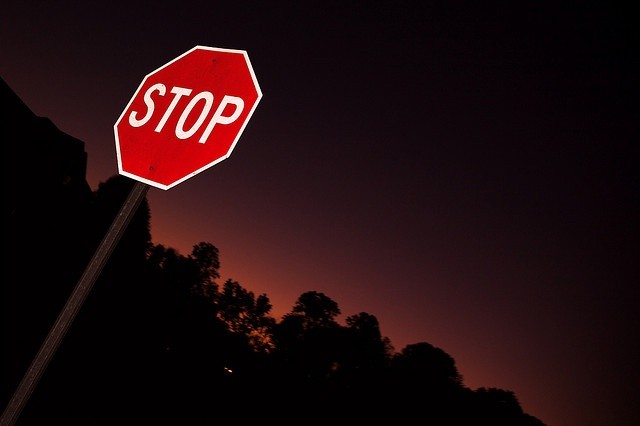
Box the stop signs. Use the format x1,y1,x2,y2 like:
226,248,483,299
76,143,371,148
112,45,264,193
